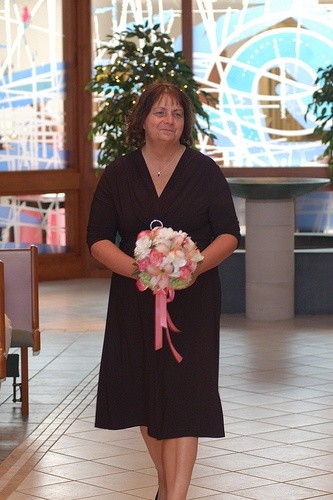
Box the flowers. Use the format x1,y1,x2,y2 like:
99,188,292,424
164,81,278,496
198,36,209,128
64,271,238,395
131,226,205,294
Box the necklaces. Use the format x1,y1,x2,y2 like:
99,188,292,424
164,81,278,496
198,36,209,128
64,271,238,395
143,151,180,178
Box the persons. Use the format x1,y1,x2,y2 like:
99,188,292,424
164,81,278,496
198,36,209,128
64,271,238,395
86,81,242,500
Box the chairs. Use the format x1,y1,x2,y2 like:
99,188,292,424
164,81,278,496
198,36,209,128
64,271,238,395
0,243,41,417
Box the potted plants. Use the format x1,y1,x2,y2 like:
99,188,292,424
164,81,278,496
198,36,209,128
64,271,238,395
82,18,217,174
301,64,333,186
